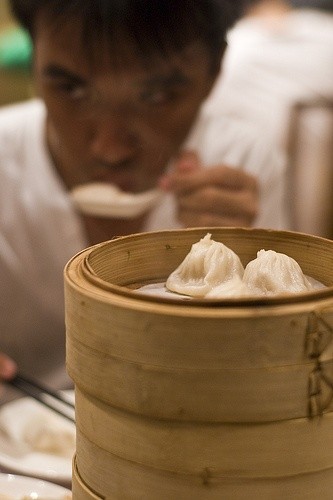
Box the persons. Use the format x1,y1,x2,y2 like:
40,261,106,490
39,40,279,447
1,0,290,401
224,0,333,239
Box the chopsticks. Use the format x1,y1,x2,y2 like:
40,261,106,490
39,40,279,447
5,371,75,425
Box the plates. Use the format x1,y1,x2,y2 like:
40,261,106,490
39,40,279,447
0,475,72,500
1,390,77,485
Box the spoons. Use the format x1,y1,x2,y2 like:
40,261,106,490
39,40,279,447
69,171,190,220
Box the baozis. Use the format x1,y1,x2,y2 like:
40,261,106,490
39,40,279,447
166,232,314,300
